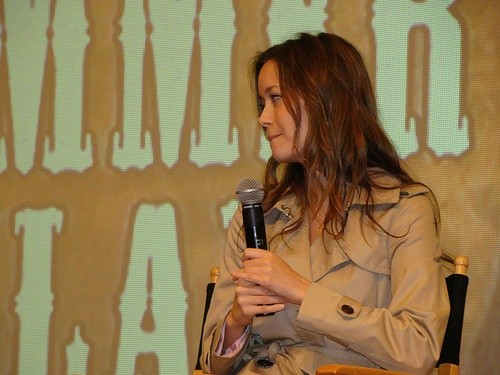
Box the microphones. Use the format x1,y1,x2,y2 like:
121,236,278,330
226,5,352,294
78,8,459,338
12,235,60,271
236,177,275,317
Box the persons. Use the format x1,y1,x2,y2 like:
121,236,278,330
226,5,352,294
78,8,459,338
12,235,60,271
199,31,451,375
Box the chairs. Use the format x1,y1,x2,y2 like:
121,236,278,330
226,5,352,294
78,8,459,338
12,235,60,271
192,243,470,375
